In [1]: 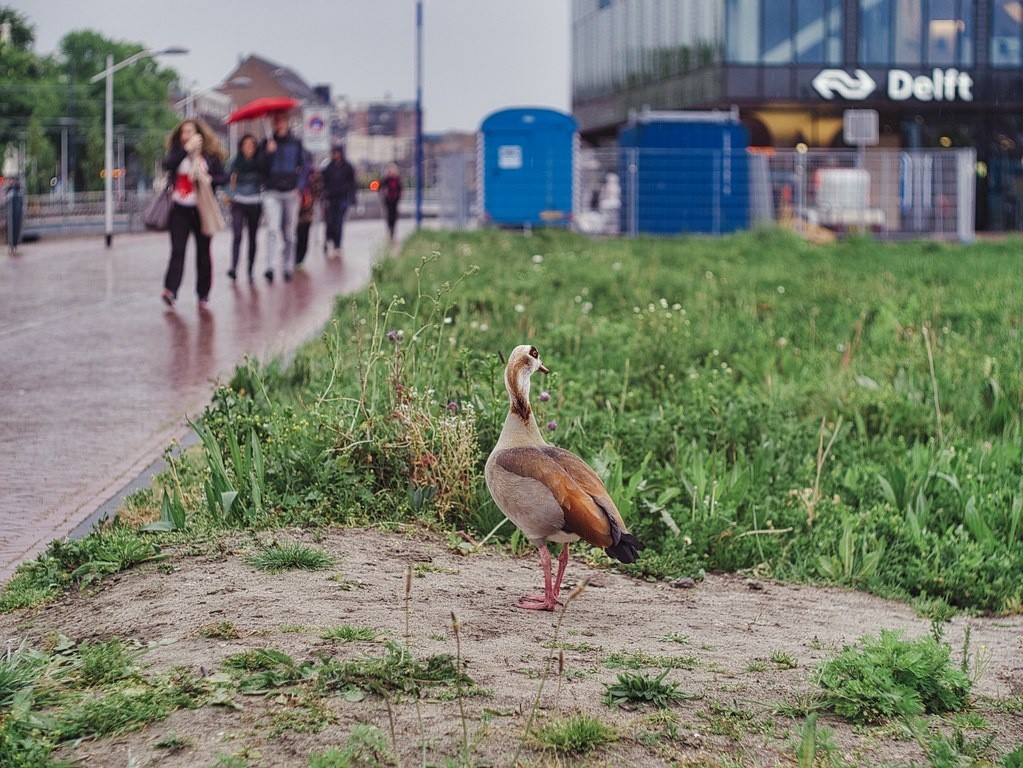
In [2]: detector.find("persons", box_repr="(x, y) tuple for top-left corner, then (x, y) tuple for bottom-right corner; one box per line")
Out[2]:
(161, 119), (229, 310)
(224, 135), (265, 280)
(258, 110), (307, 282)
(295, 153), (323, 271)
(381, 160), (401, 237)
(321, 144), (357, 249)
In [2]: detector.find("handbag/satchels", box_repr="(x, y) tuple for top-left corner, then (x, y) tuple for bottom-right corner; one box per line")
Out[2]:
(144, 187), (173, 231)
(194, 168), (226, 236)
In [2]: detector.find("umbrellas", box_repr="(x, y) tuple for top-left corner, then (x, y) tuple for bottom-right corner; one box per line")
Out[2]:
(225, 97), (299, 141)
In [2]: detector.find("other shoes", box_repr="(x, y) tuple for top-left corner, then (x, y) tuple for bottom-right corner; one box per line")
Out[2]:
(264, 269), (273, 283)
(163, 292), (177, 308)
(227, 267), (237, 280)
(199, 297), (208, 311)
(283, 271), (293, 283)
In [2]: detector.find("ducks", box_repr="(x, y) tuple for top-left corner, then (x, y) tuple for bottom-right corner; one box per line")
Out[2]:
(486, 345), (647, 612)
